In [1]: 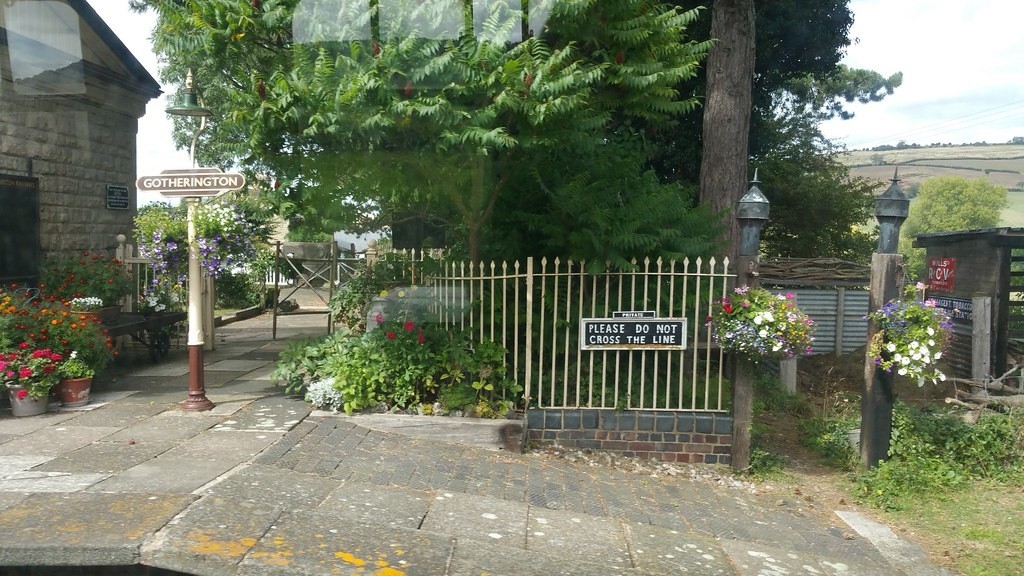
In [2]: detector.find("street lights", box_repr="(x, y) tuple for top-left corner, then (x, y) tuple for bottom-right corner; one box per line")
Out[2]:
(165, 68), (215, 413)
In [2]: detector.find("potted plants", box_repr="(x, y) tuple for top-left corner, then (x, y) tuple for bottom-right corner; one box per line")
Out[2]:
(53, 356), (96, 408)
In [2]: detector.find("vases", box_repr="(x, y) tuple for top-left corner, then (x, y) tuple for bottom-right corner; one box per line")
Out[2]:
(5, 378), (51, 417)
(98, 304), (125, 325)
(70, 308), (104, 326)
(140, 307), (165, 319)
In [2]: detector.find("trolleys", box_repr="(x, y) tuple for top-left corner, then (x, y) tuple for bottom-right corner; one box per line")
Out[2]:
(104, 310), (187, 364)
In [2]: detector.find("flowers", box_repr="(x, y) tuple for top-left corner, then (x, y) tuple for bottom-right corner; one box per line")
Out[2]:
(69, 296), (105, 312)
(0, 341), (64, 401)
(863, 280), (961, 387)
(705, 282), (818, 363)
(133, 278), (185, 313)
(65, 252), (138, 306)
